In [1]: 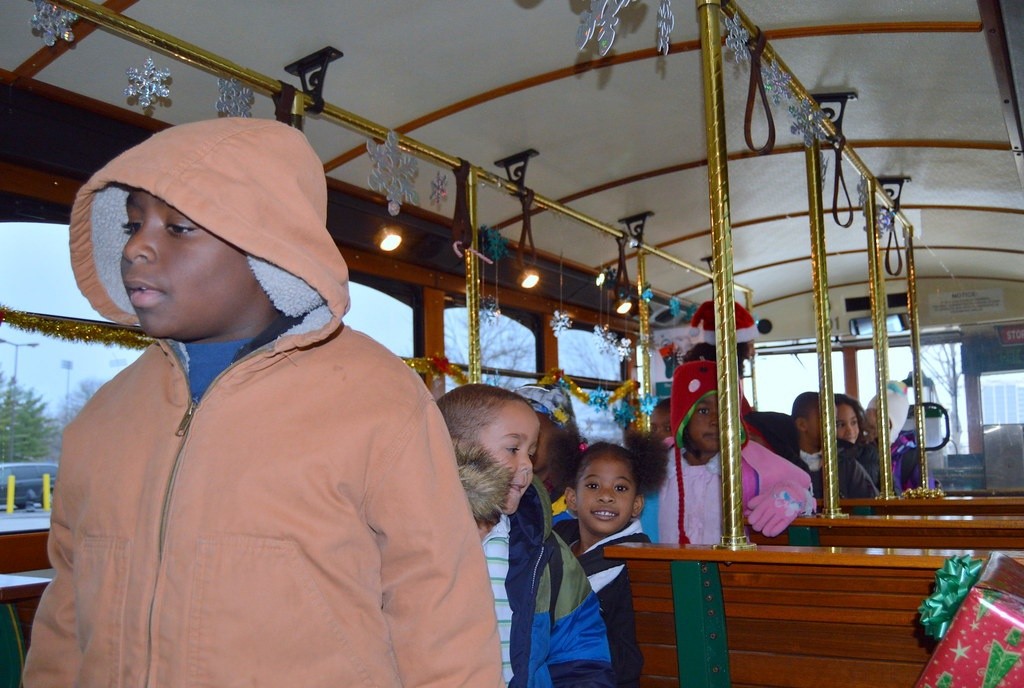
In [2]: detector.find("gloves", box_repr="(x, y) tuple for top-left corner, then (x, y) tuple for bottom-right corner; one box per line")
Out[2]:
(747, 486), (812, 537)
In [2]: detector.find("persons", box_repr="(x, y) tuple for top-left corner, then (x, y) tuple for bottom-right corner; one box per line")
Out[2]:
(689, 299), (758, 414)
(550, 440), (652, 688)
(511, 382), (587, 523)
(642, 397), (674, 441)
(789, 389), (879, 501)
(834, 393), (902, 497)
(641, 361), (815, 546)
(865, 381), (936, 493)
(435, 381), (611, 688)
(19, 118), (506, 688)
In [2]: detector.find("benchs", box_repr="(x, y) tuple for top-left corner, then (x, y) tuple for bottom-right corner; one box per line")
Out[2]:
(603, 542), (1024, 688)
(817, 495), (1024, 522)
(744, 513), (1024, 551)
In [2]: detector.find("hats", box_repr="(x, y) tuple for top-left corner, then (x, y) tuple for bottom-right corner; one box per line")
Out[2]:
(868, 379), (910, 445)
(670, 361), (749, 547)
(515, 383), (576, 432)
